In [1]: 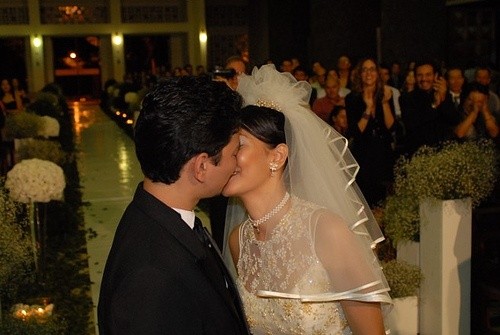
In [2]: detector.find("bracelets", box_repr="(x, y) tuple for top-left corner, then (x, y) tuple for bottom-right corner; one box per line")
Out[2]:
(484, 114), (496, 122)
(361, 112), (372, 120)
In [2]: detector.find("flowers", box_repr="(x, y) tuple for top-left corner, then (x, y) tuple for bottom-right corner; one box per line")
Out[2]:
(37, 116), (60, 140)
(4, 157), (67, 271)
(125, 92), (140, 105)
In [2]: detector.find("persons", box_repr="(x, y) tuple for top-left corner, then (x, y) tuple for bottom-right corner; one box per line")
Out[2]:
(122, 55), (500, 230)
(98, 76), (252, 335)
(0, 76), (26, 176)
(220, 64), (399, 335)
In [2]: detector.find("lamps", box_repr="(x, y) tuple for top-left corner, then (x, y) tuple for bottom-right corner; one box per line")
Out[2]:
(13, 303), (54, 325)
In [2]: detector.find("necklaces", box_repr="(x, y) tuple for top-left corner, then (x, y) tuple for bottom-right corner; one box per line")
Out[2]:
(248, 192), (290, 233)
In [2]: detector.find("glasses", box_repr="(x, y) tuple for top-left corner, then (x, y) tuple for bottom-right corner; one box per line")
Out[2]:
(362, 67), (377, 72)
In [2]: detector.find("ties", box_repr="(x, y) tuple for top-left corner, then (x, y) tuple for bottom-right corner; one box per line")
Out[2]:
(193, 216), (246, 323)
(454, 96), (459, 107)
